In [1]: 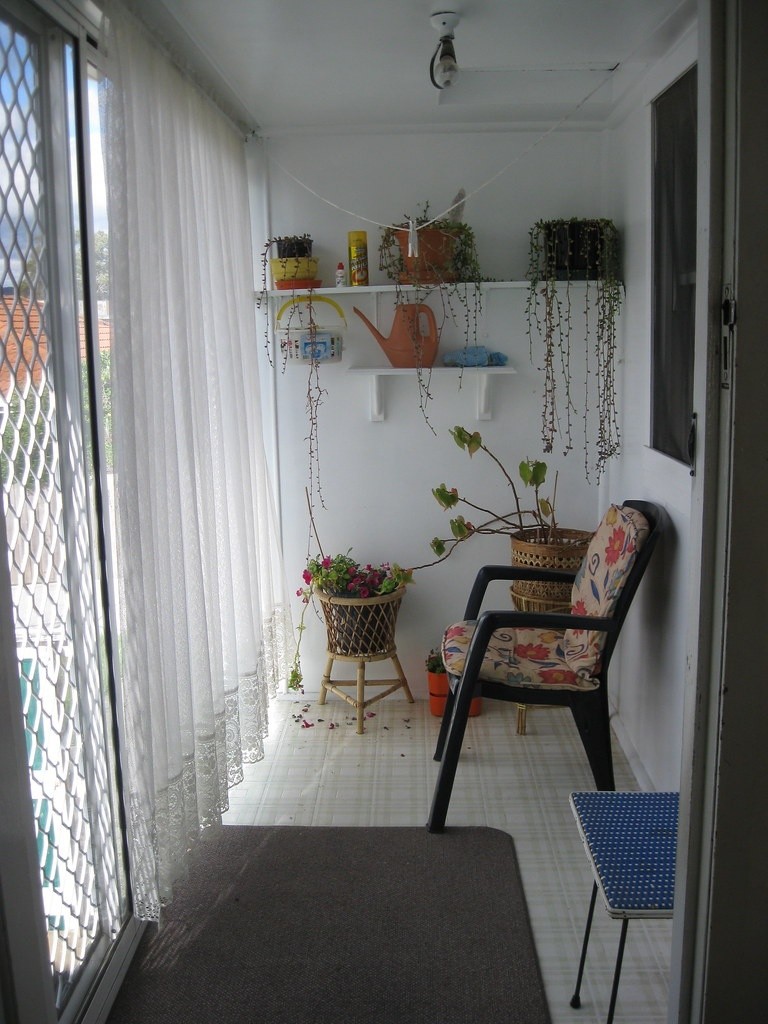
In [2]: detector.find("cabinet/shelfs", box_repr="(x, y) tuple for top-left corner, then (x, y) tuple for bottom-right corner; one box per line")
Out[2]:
(254, 278), (624, 423)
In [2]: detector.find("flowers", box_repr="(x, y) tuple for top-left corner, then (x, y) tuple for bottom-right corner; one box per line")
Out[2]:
(287, 546), (416, 690)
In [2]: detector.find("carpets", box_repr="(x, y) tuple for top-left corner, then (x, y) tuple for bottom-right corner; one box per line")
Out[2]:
(106, 825), (552, 1024)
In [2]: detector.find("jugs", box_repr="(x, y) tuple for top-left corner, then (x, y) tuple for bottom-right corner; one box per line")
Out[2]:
(350, 303), (438, 368)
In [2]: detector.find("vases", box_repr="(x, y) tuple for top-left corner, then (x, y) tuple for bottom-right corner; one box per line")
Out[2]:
(327, 594), (389, 654)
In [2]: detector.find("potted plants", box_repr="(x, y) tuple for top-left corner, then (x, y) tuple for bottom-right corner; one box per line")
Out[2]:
(425, 647), (481, 717)
(525, 216), (621, 485)
(256, 233), (328, 625)
(378, 200), (515, 437)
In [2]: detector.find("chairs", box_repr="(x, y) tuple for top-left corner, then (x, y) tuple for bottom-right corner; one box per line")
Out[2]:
(424, 500), (663, 833)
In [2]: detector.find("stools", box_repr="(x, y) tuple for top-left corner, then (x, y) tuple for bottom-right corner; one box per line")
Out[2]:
(568, 790), (679, 1024)
